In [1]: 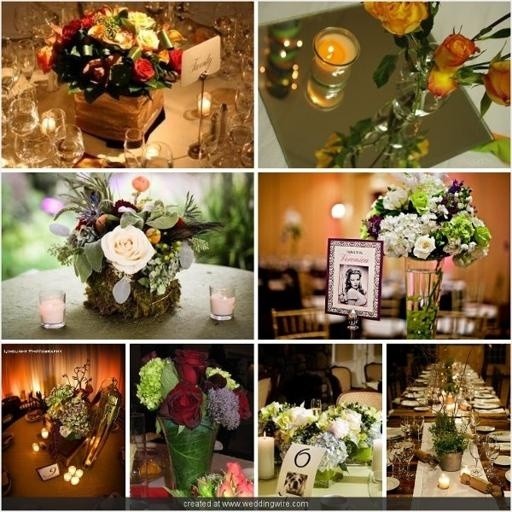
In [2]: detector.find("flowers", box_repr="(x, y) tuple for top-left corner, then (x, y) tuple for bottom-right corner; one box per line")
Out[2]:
(260, 399), (380, 484)
(39, 5), (184, 95)
(359, 171), (491, 270)
(41, 172), (225, 321)
(134, 347), (250, 428)
(311, 0), (508, 170)
(44, 360), (106, 441)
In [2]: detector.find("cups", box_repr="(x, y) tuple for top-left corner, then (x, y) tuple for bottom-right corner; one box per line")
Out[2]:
(209, 284), (237, 322)
(142, 2), (192, 33)
(303, 73), (345, 113)
(400, 400), (418, 406)
(304, 26), (361, 86)
(142, 140), (174, 168)
(414, 363), (434, 384)
(413, 406), (429, 411)
(405, 392), (422, 398)
(39, 289), (67, 330)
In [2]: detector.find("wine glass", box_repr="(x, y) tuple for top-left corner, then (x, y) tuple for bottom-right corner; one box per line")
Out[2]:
(402, 444), (415, 481)
(387, 445), (398, 478)
(486, 434), (498, 443)
(469, 441), (485, 473)
(400, 415), (414, 442)
(1, 2), (75, 98)
(39, 107), (67, 141)
(311, 399), (322, 416)
(198, 7), (254, 168)
(413, 415), (425, 443)
(53, 123), (85, 168)
(6, 98), (39, 137)
(484, 443), (500, 479)
(393, 442), (405, 473)
(13, 125), (53, 168)
(129, 411), (148, 488)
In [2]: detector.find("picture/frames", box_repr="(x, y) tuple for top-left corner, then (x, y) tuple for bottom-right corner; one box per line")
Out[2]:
(326, 238), (383, 322)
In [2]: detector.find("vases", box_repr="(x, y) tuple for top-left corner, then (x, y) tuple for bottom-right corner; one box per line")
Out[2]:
(43, 425), (87, 464)
(160, 414), (218, 497)
(71, 86), (166, 148)
(402, 270), (442, 341)
(384, 101), (426, 166)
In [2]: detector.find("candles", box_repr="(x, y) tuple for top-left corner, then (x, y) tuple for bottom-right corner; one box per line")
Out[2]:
(437, 473), (450, 490)
(198, 92), (211, 115)
(258, 430), (276, 479)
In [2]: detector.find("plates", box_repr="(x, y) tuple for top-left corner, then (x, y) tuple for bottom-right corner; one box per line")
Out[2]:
(460, 363), (485, 384)
(489, 430), (510, 442)
(476, 425), (496, 432)
(489, 442), (510, 452)
(472, 403), (500, 409)
(505, 469), (511, 482)
(387, 477), (400, 492)
(471, 385), (500, 403)
(386, 426), (410, 438)
(493, 455), (511, 465)
(406, 386), (427, 392)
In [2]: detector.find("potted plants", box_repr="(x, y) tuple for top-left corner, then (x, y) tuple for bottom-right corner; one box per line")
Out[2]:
(429, 411), (477, 470)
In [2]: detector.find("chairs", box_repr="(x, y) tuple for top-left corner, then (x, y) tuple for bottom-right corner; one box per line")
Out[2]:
(486, 363), (494, 386)
(387, 387), (393, 409)
(433, 310), (465, 339)
(336, 391), (380, 412)
(500, 378), (509, 406)
(331, 366), (352, 390)
(395, 380), (401, 397)
(270, 307), (330, 339)
(365, 362), (381, 383)
(360, 299), (400, 337)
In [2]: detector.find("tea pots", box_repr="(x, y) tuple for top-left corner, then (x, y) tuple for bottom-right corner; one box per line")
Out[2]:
(79, 377), (123, 471)
